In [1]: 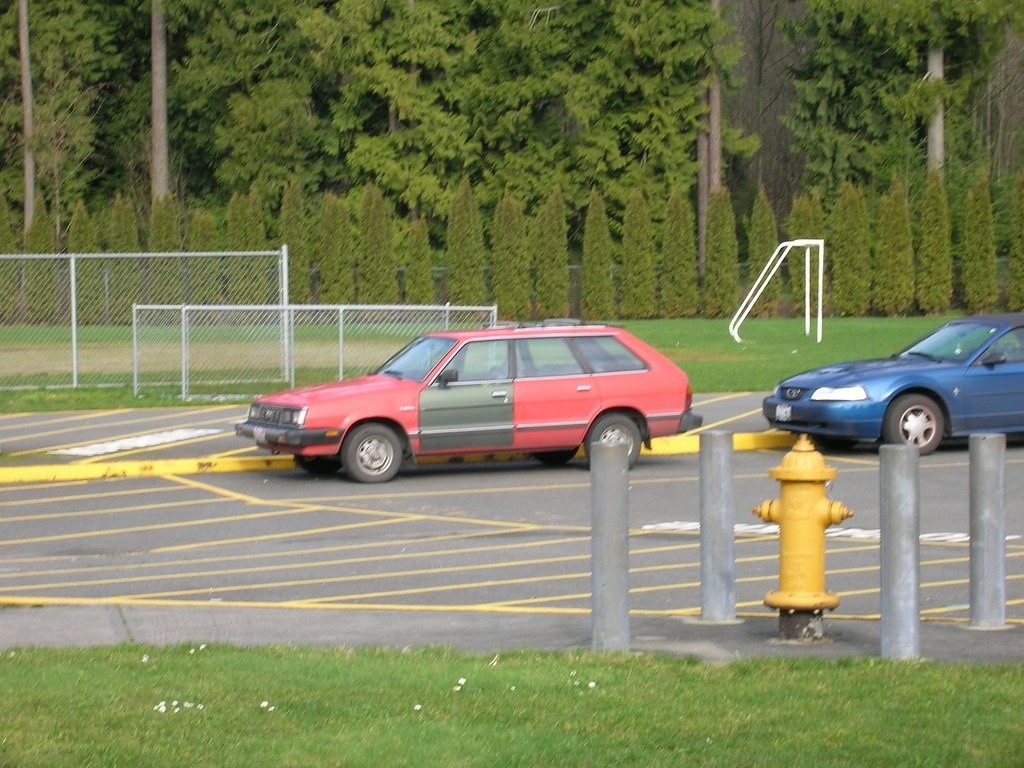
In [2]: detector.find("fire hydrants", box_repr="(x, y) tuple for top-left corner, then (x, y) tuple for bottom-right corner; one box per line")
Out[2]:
(747, 430), (854, 640)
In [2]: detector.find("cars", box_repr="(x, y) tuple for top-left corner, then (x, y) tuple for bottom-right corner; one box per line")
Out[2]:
(761, 313), (1024, 456)
(238, 324), (703, 482)
